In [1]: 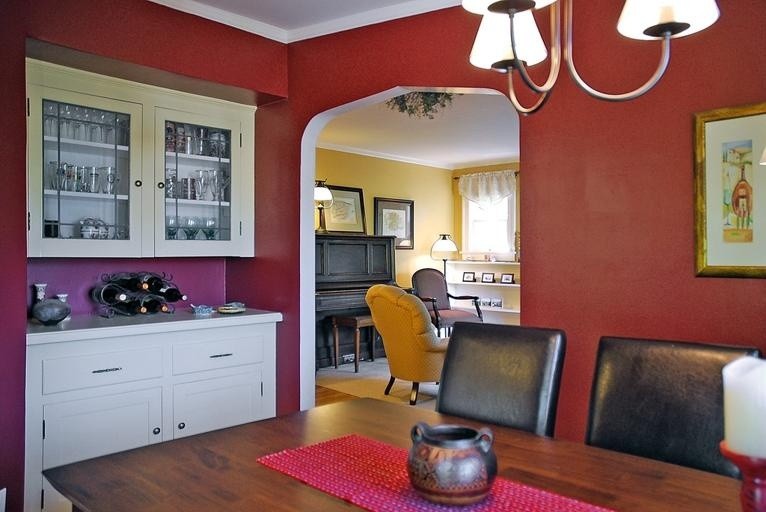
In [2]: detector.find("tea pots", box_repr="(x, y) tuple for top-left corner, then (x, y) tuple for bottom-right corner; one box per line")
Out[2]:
(80, 216), (108, 239)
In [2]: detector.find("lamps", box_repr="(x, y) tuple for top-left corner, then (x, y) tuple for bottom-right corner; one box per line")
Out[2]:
(462, 0), (721, 115)
(314, 180), (333, 234)
(430, 234), (459, 276)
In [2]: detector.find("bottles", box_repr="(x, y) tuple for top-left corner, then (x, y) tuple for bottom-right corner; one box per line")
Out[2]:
(166, 216), (222, 239)
(89, 273), (190, 314)
(33, 282), (72, 322)
(165, 125), (227, 157)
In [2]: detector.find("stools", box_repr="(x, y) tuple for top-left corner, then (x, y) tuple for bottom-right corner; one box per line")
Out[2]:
(332, 311), (376, 373)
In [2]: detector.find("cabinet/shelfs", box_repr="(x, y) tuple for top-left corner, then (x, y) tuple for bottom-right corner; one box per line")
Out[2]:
(24, 56), (255, 260)
(24, 323), (278, 512)
(447, 261), (521, 327)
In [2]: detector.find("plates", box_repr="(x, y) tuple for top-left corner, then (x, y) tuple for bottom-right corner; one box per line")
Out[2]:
(192, 306), (247, 314)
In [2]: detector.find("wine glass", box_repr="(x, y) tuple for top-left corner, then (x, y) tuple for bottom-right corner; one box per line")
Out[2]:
(43, 102), (130, 146)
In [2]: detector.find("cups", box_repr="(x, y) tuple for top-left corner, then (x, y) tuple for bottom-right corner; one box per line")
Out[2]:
(45, 219), (59, 238)
(166, 169), (224, 202)
(51, 161), (120, 195)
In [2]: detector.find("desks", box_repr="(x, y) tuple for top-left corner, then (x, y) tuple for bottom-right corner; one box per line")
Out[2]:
(41, 395), (742, 512)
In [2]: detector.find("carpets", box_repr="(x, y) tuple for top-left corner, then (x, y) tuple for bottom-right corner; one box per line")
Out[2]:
(315, 356), (438, 405)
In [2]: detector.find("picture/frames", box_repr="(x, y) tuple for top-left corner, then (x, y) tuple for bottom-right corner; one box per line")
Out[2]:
(500, 274), (515, 284)
(481, 273), (495, 283)
(693, 101), (766, 279)
(322, 184), (368, 236)
(463, 272), (476, 282)
(374, 197), (414, 250)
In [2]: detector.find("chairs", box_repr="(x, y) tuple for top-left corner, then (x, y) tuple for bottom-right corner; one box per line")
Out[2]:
(435, 318), (566, 441)
(585, 335), (762, 483)
(365, 283), (450, 406)
(410, 268), (483, 338)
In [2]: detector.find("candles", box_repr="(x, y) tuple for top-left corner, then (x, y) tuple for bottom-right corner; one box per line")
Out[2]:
(722, 355), (766, 458)
